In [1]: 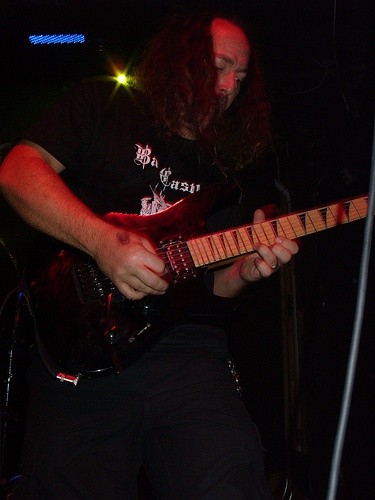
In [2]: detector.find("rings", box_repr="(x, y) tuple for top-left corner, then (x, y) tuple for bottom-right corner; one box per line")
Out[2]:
(271, 263), (278, 269)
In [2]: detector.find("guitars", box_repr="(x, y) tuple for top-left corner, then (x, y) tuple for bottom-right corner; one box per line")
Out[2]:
(21, 191), (375, 388)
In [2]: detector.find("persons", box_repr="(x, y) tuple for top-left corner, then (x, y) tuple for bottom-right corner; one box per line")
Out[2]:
(1, 4), (300, 500)
